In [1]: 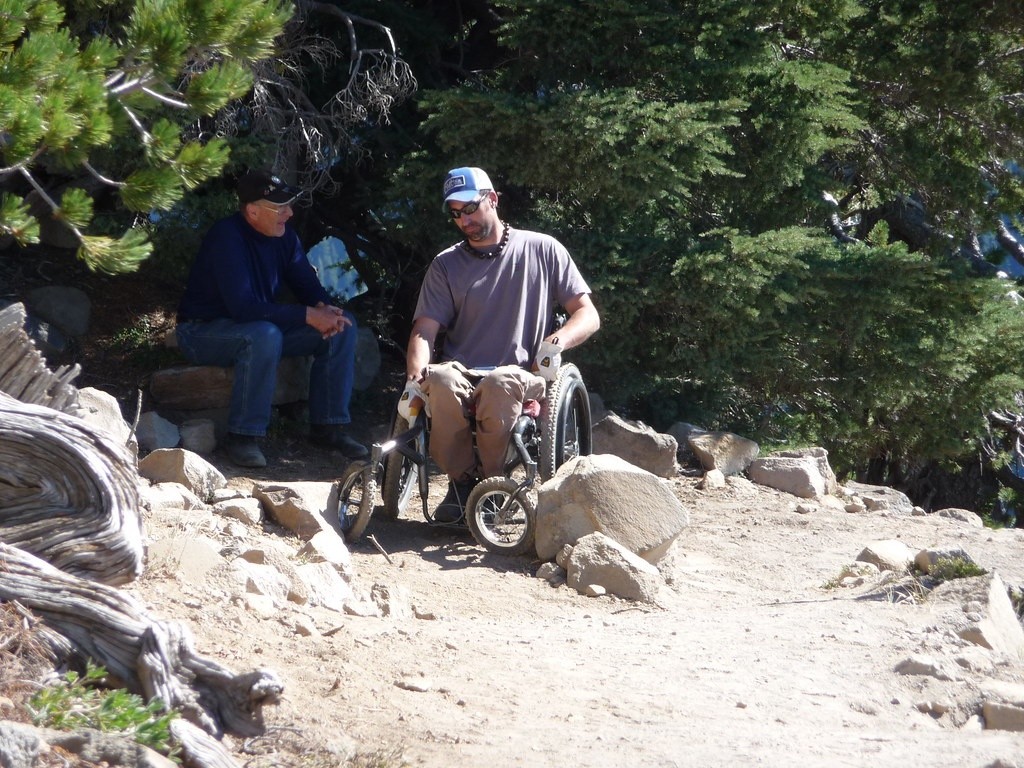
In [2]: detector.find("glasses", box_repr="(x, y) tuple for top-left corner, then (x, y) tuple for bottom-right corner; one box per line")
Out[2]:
(256, 203), (288, 215)
(450, 193), (488, 219)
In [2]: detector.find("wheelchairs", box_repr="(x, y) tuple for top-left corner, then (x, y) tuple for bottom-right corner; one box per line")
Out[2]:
(337, 310), (595, 558)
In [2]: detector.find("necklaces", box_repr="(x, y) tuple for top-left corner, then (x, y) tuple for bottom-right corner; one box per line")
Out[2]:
(463, 220), (510, 260)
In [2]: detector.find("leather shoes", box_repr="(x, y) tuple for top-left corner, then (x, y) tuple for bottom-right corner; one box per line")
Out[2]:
(309, 424), (369, 457)
(226, 432), (267, 467)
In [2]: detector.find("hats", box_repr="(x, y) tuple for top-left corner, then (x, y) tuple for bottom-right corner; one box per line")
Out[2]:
(441, 166), (494, 213)
(237, 169), (305, 205)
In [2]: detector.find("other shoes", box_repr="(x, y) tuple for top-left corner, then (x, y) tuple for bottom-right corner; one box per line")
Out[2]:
(481, 492), (504, 523)
(434, 464), (484, 522)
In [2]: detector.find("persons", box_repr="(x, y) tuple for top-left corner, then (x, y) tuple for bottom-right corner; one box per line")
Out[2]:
(399, 165), (602, 525)
(174, 170), (370, 468)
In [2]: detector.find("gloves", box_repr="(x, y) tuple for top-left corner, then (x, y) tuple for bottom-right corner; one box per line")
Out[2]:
(397, 376), (431, 430)
(530, 337), (563, 381)
